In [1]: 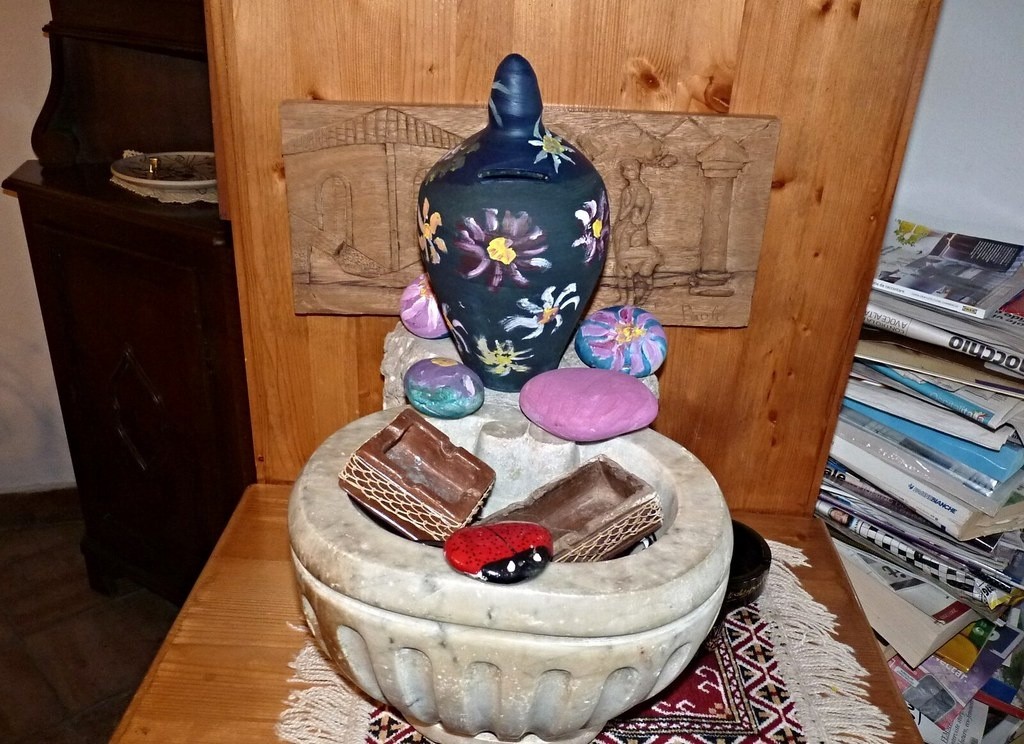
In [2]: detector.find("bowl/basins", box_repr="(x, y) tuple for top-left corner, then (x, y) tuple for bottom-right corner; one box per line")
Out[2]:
(717, 519), (770, 627)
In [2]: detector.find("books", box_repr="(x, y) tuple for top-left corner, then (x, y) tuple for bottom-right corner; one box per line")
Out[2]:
(812, 214), (1024, 744)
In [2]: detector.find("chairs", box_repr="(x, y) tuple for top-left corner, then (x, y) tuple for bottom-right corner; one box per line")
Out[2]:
(109, 0), (946, 744)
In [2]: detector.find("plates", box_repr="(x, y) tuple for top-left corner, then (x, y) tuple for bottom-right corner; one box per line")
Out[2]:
(111, 152), (217, 190)
